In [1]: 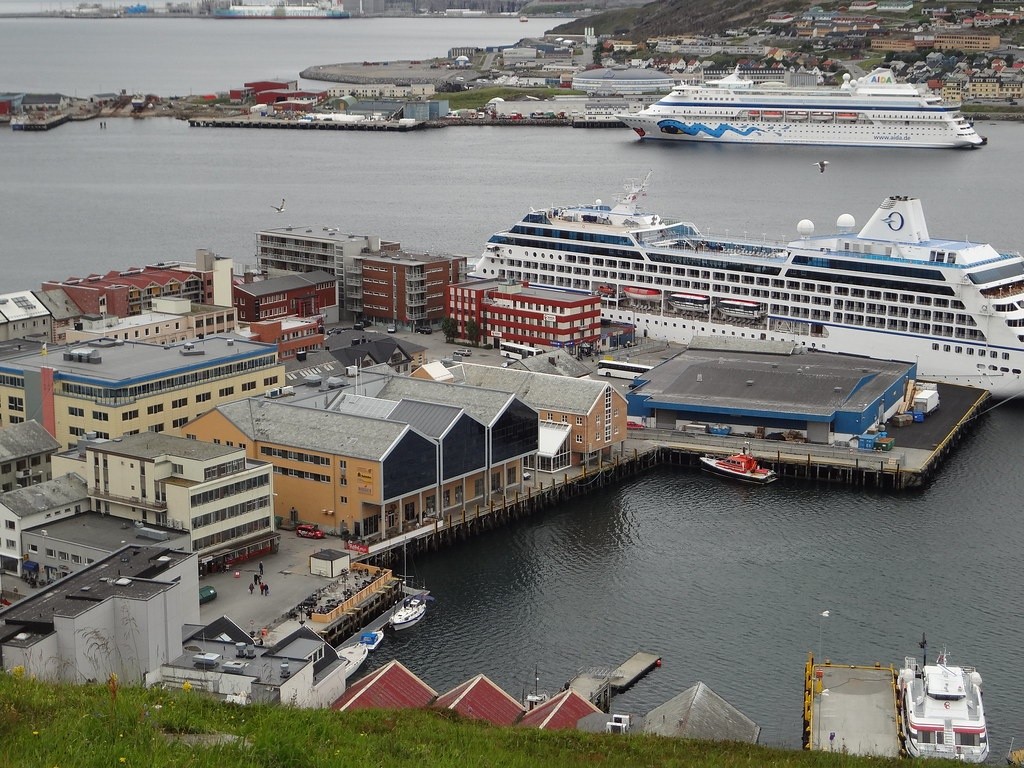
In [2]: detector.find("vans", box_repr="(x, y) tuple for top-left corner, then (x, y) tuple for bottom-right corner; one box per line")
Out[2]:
(387, 324), (397, 333)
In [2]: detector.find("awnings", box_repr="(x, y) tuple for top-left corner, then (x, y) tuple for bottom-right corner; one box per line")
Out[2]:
(22, 561), (39, 573)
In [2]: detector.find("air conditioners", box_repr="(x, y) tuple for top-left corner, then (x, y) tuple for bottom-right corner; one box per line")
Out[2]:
(605, 721), (626, 735)
(613, 714), (629, 729)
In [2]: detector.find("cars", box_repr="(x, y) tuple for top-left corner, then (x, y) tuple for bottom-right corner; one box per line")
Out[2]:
(357, 320), (372, 328)
(626, 421), (645, 430)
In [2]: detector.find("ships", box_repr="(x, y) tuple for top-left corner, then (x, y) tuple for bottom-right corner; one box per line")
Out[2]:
(613, 63), (984, 150)
(215, 4), (351, 20)
(465, 168), (1024, 401)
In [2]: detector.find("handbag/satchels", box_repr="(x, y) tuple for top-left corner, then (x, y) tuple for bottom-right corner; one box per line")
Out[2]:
(248, 589), (251, 593)
(266, 591), (269, 594)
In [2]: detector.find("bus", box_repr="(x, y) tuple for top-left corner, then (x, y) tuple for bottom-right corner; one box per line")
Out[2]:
(500, 341), (543, 361)
(597, 360), (654, 380)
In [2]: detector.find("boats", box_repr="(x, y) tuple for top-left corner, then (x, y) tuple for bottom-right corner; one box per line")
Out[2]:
(597, 285), (616, 295)
(761, 112), (783, 118)
(810, 112), (833, 120)
(336, 643), (369, 680)
(836, 113), (858, 120)
(786, 111), (807, 119)
(667, 293), (710, 313)
(360, 630), (384, 653)
(715, 300), (761, 320)
(623, 286), (661, 301)
(390, 599), (427, 631)
(900, 649), (990, 761)
(748, 111), (759, 117)
(699, 452), (778, 487)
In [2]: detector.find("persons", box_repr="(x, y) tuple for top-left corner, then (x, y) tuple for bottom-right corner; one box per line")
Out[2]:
(249, 627), (268, 646)
(416, 510), (428, 524)
(341, 526), (350, 550)
(217, 562), (232, 573)
(295, 567), (379, 620)
(14, 577), (53, 593)
(248, 560), (268, 596)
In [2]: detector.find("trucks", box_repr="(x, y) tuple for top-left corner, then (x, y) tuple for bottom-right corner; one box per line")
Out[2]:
(914, 390), (941, 417)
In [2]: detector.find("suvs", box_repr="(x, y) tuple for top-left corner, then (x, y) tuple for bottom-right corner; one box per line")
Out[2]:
(453, 348), (472, 357)
(415, 327), (432, 335)
(296, 524), (324, 540)
